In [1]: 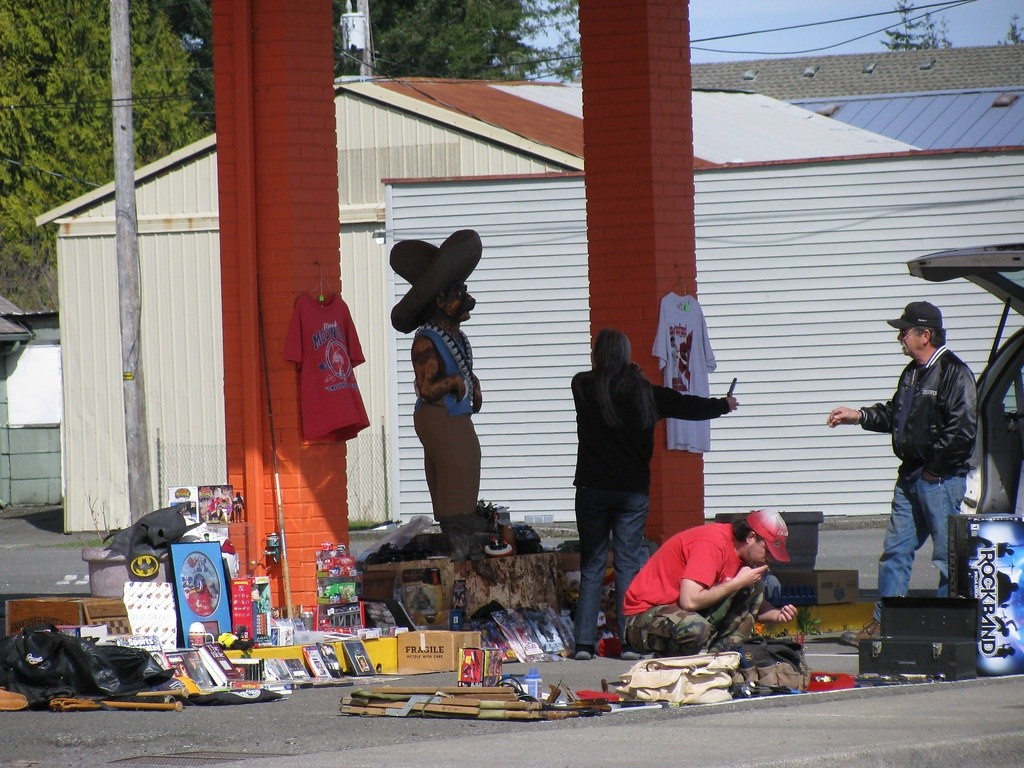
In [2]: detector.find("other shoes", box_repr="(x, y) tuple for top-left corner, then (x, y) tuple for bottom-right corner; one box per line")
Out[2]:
(619, 647), (655, 659)
(574, 644), (597, 659)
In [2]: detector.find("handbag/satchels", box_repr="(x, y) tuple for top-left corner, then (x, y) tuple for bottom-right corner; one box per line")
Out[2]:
(1, 623), (183, 701)
(614, 632), (812, 705)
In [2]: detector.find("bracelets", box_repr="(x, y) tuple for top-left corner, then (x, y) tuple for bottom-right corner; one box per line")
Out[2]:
(854, 410), (860, 425)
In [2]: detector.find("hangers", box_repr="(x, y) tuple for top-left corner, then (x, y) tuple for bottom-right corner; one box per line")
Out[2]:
(307, 260), (336, 296)
(671, 264), (690, 296)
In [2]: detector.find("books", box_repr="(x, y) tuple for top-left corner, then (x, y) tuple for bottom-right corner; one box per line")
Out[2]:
(197, 644), (242, 686)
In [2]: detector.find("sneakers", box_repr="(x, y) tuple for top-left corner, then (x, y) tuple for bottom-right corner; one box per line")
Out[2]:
(840, 618), (881, 647)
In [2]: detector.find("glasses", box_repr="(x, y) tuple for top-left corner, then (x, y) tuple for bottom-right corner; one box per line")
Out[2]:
(902, 329), (914, 336)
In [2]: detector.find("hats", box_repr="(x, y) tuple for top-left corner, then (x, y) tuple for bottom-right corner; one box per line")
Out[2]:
(887, 300), (942, 328)
(748, 508), (791, 561)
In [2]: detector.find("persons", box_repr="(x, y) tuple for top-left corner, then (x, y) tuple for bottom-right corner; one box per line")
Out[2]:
(388, 229), (484, 517)
(357, 657), (370, 671)
(620, 510), (798, 659)
(826, 300), (978, 637)
(571, 330), (739, 660)
(319, 644), (342, 677)
(274, 659), (284, 675)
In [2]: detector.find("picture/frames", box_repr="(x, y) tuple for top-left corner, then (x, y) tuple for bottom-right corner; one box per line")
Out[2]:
(167, 541), (234, 648)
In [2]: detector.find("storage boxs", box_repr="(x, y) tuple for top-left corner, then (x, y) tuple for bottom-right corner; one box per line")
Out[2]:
(397, 630), (481, 672)
(858, 596), (981, 680)
(4, 597), (123, 645)
(414, 609), (463, 631)
(317, 557), (383, 639)
(168, 484), (235, 525)
(230, 576), (295, 647)
(458, 646), (502, 687)
(294, 631), (324, 644)
(769, 569), (859, 606)
(802, 642), (860, 675)
(947, 514), (1024, 677)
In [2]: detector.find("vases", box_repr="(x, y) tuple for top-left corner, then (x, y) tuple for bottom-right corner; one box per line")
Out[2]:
(715, 512), (824, 569)
(82, 547), (173, 597)
